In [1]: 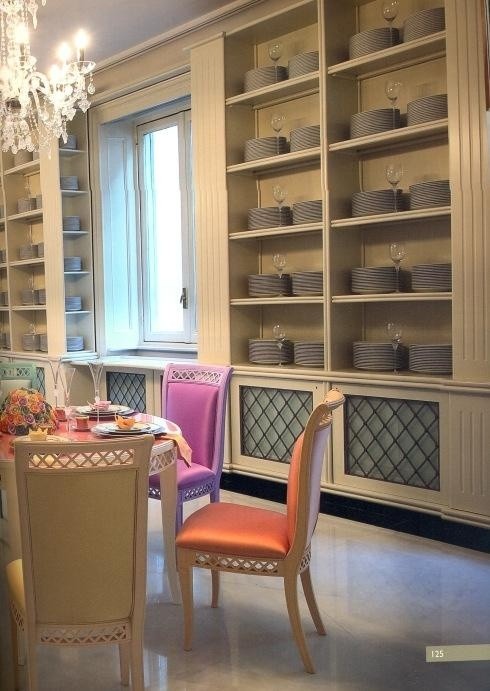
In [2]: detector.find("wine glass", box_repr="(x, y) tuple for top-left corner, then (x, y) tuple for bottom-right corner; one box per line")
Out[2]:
(49, 357), (104, 430)
(268, 42), (288, 369)
(384, 1), (407, 374)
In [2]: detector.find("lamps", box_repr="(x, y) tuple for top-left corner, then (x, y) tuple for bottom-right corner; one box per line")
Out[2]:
(0, 0), (96, 155)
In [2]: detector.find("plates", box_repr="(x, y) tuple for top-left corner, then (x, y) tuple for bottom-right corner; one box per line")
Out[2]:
(8, 434), (70, 452)
(246, 337), (326, 368)
(71, 404), (136, 418)
(347, 179), (451, 215)
(242, 125), (324, 161)
(345, 263), (454, 294)
(348, 95), (447, 141)
(0, 135), (85, 354)
(246, 270), (325, 298)
(354, 340), (451, 374)
(342, 6), (446, 59)
(237, 200), (325, 229)
(243, 50), (320, 91)
(94, 421), (167, 436)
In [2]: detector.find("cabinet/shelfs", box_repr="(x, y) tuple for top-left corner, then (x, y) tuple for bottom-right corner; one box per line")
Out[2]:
(228, 375), (447, 514)
(0, 109), (97, 361)
